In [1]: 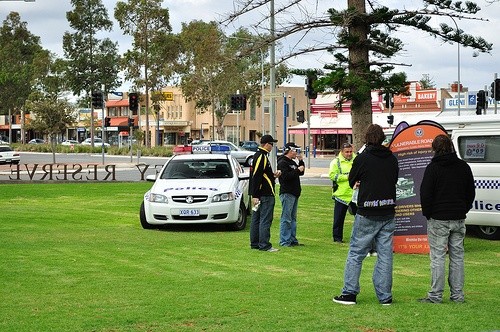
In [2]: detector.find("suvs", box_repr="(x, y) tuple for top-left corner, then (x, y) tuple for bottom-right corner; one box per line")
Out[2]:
(201, 141), (257, 168)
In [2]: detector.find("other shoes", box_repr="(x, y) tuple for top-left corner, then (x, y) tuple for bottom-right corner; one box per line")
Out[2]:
(295, 243), (304, 246)
(418, 298), (434, 304)
(286, 244), (293, 247)
(266, 247), (279, 252)
(334, 240), (343, 243)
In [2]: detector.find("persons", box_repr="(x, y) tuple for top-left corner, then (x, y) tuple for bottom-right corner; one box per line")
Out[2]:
(420, 133), (475, 302)
(329, 143), (357, 243)
(277, 143), (306, 246)
(248, 135), (281, 251)
(332, 124), (399, 305)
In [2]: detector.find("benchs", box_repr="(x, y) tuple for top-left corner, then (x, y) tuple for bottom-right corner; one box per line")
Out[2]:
(191, 166), (216, 176)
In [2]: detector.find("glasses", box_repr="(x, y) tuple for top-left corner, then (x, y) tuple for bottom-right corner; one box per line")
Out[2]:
(265, 143), (273, 146)
(292, 150), (297, 152)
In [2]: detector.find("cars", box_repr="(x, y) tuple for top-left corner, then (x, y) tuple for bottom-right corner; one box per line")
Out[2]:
(82, 137), (110, 147)
(0, 146), (20, 164)
(139, 146), (251, 231)
(241, 141), (259, 152)
(28, 139), (45, 144)
(61, 140), (80, 145)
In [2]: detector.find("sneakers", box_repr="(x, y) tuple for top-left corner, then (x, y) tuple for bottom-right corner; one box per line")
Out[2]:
(333, 294), (356, 304)
(382, 298), (392, 305)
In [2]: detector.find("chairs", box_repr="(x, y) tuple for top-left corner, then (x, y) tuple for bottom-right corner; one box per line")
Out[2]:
(176, 164), (190, 174)
(214, 164), (228, 176)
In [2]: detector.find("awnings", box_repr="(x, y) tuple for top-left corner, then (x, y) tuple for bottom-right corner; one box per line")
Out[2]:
(86, 117), (138, 131)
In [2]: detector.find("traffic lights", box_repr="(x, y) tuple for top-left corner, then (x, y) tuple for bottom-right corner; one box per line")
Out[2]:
(129, 93), (138, 110)
(230, 95), (248, 110)
(491, 78), (500, 101)
(476, 90), (485, 114)
(297, 110), (305, 122)
(128, 118), (134, 126)
(92, 92), (103, 109)
(307, 75), (318, 99)
(387, 115), (394, 124)
(105, 117), (111, 127)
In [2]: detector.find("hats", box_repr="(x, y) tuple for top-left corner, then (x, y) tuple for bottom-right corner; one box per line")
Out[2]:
(260, 135), (278, 143)
(284, 142), (301, 150)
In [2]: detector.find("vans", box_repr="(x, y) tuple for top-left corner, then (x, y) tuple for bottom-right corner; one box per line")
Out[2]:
(450, 128), (500, 240)
